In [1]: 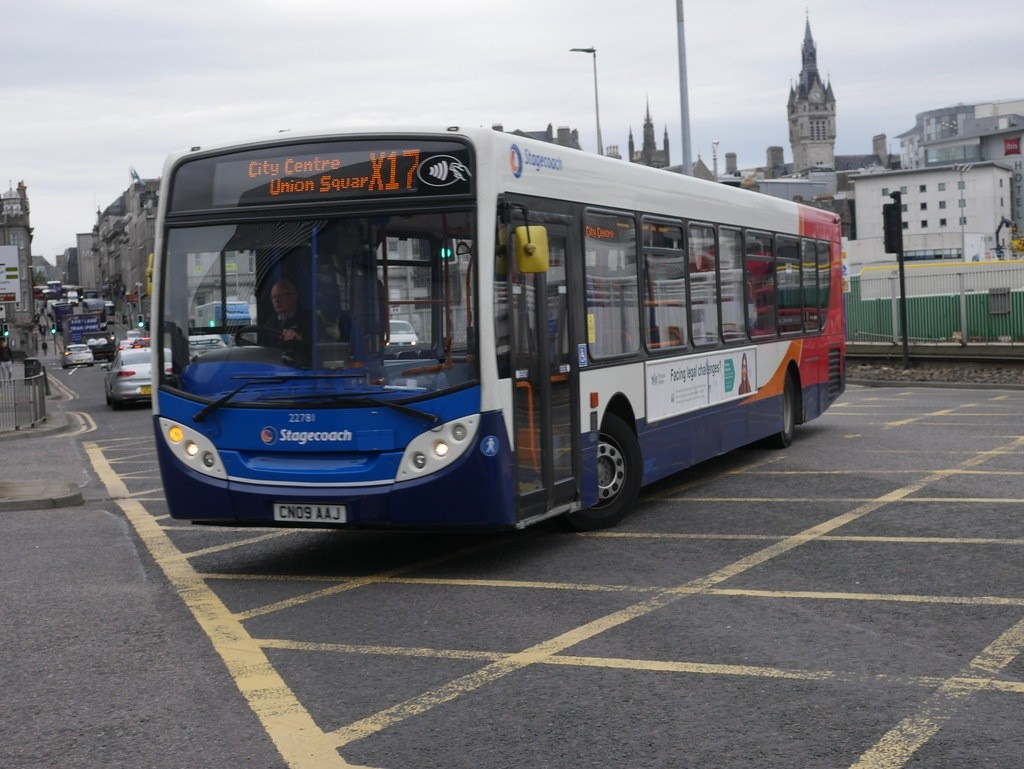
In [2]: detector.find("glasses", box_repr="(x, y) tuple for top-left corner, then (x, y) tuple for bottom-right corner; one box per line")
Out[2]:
(270, 293), (293, 298)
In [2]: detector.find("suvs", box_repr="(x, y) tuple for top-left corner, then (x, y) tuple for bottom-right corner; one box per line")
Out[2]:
(80, 332), (115, 362)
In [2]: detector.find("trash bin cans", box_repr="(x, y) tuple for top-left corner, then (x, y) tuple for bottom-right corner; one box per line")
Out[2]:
(123, 316), (127, 324)
(24, 358), (41, 385)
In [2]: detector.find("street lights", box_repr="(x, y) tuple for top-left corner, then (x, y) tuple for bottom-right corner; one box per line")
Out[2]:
(569, 46), (604, 155)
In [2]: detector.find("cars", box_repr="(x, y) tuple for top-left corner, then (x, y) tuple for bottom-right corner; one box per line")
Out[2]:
(104, 329), (177, 410)
(60, 344), (95, 368)
(384, 319), (419, 346)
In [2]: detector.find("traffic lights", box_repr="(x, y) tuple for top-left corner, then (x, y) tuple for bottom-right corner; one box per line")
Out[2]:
(3, 323), (9, 337)
(137, 314), (144, 329)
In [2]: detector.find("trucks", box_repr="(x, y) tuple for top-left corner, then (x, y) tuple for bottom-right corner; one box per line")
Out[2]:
(194, 300), (253, 328)
(32, 280), (116, 348)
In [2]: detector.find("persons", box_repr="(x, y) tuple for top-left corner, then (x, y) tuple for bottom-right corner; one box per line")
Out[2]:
(346, 247), (390, 383)
(0, 340), (15, 386)
(261, 279), (323, 365)
(42, 342), (48, 356)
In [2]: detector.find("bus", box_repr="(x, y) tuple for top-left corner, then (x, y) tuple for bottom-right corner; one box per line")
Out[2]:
(145, 130), (847, 543)
